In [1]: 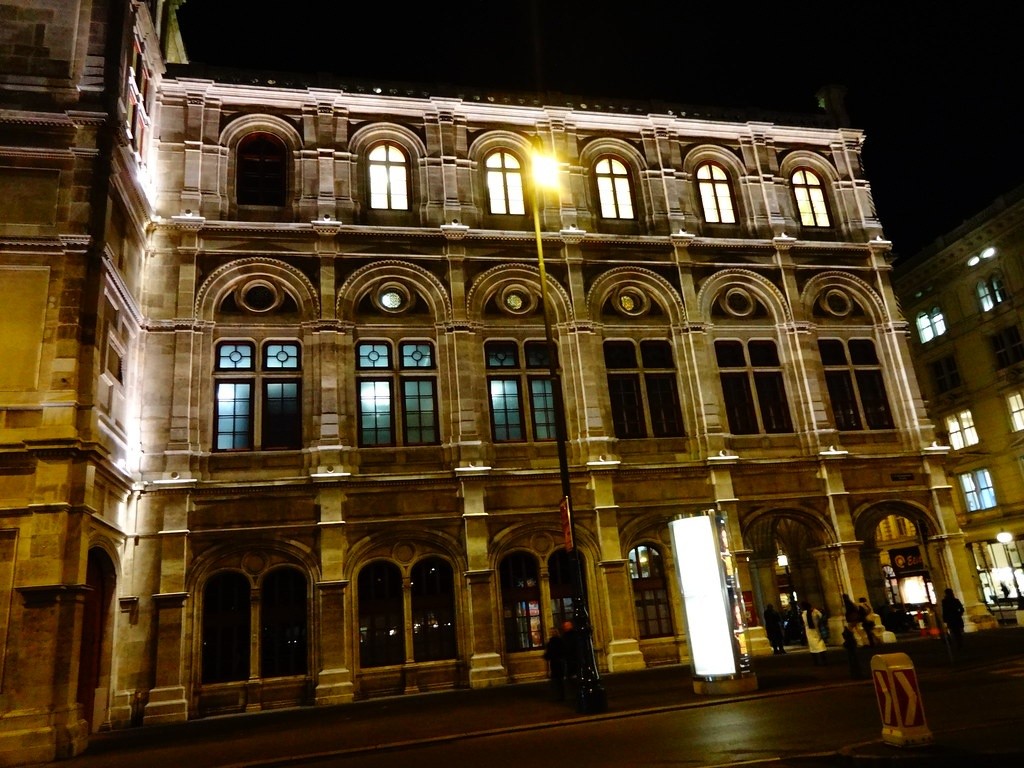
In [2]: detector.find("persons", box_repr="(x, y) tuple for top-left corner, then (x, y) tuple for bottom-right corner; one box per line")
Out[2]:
(842, 593), (875, 648)
(801, 602), (829, 665)
(1001, 584), (1009, 598)
(941, 588), (964, 656)
(764, 604), (787, 654)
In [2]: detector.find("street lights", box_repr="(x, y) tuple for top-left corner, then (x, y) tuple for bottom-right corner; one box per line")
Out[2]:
(996, 531), (1024, 610)
(524, 151), (607, 715)
